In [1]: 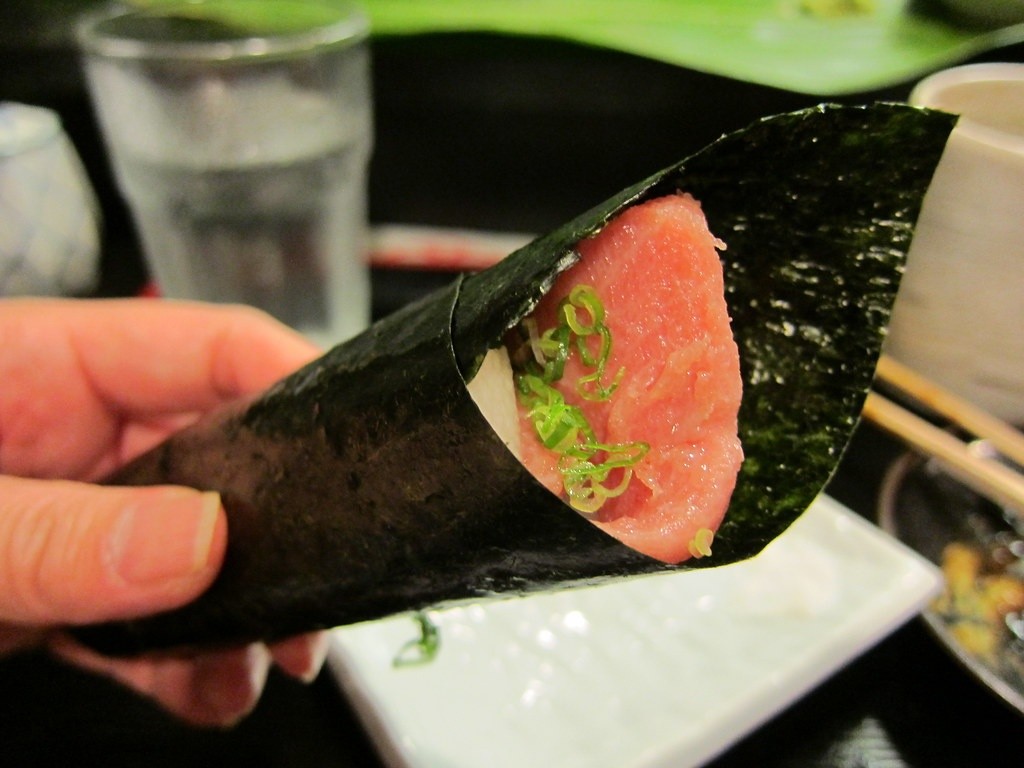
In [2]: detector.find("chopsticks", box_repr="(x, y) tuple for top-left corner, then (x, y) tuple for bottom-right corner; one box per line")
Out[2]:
(859, 351), (1023, 514)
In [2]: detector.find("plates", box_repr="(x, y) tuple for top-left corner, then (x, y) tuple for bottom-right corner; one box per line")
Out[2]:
(880, 426), (1023, 715)
(321, 490), (945, 768)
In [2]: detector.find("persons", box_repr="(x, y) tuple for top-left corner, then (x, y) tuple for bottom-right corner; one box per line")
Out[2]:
(1, 288), (336, 735)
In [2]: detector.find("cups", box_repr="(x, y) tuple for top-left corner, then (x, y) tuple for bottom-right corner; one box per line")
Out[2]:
(890, 59), (1023, 428)
(3, 98), (96, 303)
(74, 0), (377, 358)
